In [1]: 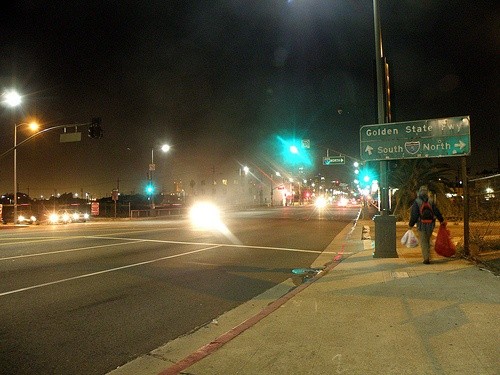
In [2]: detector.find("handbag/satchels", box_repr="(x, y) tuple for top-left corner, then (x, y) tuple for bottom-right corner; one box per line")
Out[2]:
(434, 222), (456, 258)
(401, 230), (419, 248)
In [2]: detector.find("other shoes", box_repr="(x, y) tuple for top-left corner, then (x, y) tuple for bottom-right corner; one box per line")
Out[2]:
(423, 261), (430, 264)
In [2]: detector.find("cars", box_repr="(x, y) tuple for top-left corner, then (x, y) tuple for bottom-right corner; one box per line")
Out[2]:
(15, 211), (36, 226)
(45, 208), (89, 225)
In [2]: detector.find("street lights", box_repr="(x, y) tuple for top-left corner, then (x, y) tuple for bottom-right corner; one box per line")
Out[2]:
(14, 121), (38, 224)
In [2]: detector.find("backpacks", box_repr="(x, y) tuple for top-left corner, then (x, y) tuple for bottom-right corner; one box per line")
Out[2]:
(417, 201), (435, 230)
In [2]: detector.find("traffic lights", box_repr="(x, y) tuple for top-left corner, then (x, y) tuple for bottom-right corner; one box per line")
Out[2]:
(88, 127), (96, 140)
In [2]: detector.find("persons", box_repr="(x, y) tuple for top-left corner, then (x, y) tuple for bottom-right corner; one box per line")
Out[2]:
(281, 192), (297, 207)
(408, 186), (447, 264)
